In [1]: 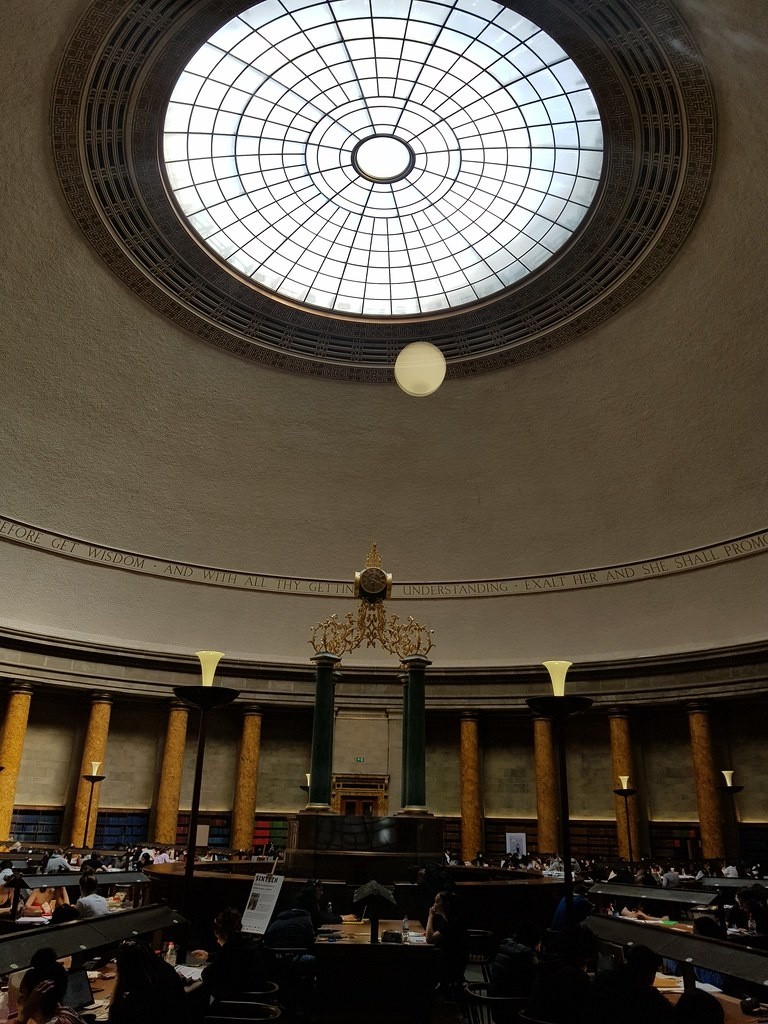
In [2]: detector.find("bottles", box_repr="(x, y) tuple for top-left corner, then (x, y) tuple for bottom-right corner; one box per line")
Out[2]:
(165, 942), (177, 967)
(402, 915), (410, 944)
(609, 903), (614, 916)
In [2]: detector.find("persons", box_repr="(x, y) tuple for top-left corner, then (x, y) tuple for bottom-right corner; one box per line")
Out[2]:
(108, 939), (194, 1024)
(0, 840), (768, 1024)
(5, 947), (89, 1024)
(190, 912), (273, 990)
(419, 891), (472, 973)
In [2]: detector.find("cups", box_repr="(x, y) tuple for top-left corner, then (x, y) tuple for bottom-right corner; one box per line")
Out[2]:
(614, 911), (619, 918)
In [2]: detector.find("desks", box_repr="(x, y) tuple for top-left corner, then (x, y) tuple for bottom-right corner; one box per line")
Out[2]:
(605, 908), (768, 1024)
(0, 861), (211, 1024)
(314, 918), (435, 1017)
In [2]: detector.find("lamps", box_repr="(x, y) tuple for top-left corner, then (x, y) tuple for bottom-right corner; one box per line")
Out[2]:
(171, 650), (242, 962)
(306, 773), (311, 786)
(718, 770), (743, 864)
(524, 660), (598, 927)
(614, 776), (635, 873)
(82, 761), (105, 848)
(394, 342), (447, 397)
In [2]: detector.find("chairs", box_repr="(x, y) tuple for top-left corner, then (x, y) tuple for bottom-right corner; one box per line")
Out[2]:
(462, 981), (558, 1024)
(204, 981), (281, 1024)
(467, 929), (496, 983)
(264, 946), (311, 1003)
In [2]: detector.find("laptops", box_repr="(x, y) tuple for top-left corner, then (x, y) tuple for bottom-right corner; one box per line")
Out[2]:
(341, 905), (367, 924)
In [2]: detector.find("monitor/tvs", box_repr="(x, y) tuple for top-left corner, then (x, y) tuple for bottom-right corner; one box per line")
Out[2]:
(61, 966), (95, 1012)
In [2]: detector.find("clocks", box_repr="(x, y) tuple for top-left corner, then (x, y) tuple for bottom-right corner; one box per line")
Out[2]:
(360, 568), (387, 594)
